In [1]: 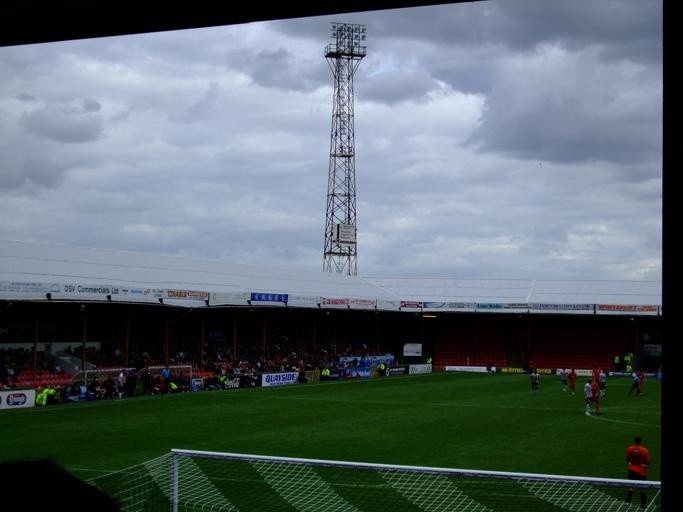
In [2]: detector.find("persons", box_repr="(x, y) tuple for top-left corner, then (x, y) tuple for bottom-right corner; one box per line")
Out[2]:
(0, 335), (389, 409)
(623, 436), (652, 512)
(529, 369), (540, 391)
(560, 351), (644, 419)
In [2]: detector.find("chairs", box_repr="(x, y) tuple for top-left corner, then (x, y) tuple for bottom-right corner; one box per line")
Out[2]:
(0, 359), (215, 391)
(430, 346), (613, 373)
